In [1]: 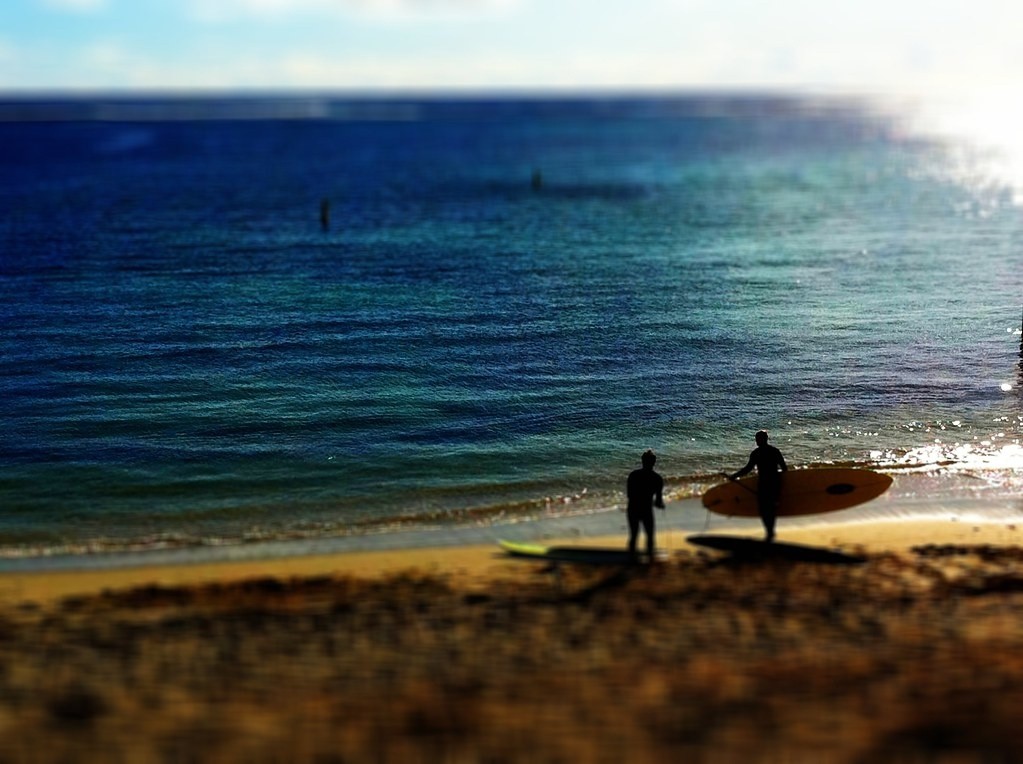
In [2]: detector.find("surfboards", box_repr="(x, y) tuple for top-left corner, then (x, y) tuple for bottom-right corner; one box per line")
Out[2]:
(703, 468), (893, 516)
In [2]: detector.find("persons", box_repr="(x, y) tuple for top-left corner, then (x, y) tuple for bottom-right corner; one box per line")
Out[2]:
(727, 431), (789, 542)
(625, 450), (667, 560)
